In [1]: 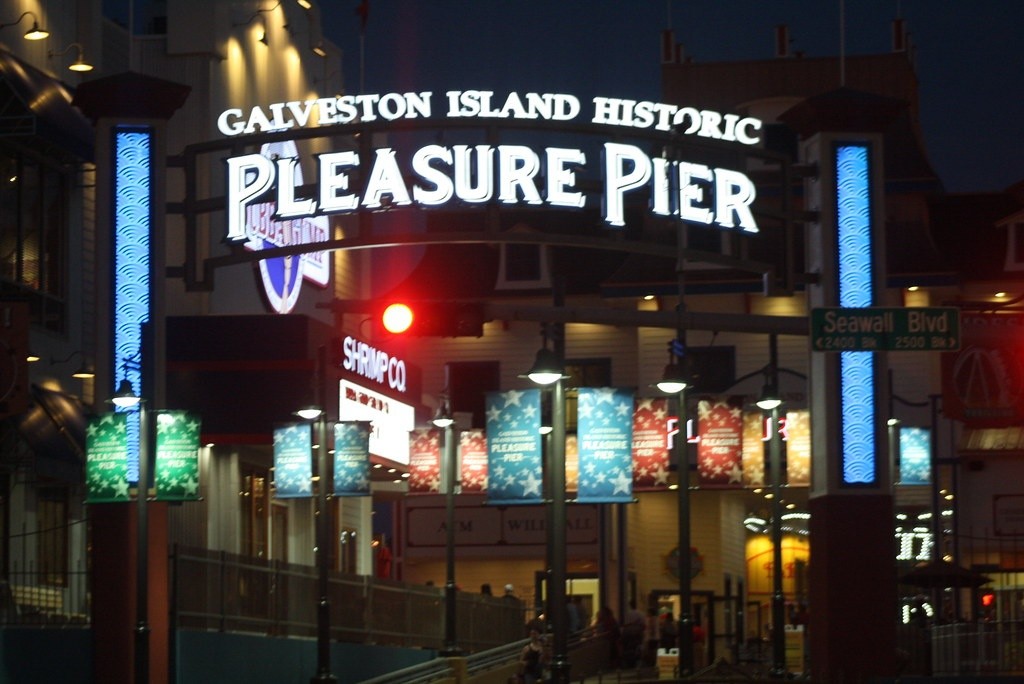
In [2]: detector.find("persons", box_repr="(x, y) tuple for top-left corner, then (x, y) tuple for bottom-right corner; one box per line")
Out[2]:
(502, 583), (518, 601)
(622, 600), (646, 672)
(787, 603), (810, 636)
(655, 604), (674, 654)
(425, 580), (434, 587)
(642, 605), (658, 664)
(565, 594), (588, 641)
(596, 605), (623, 674)
(517, 626), (549, 684)
(691, 619), (707, 673)
(480, 584), (493, 596)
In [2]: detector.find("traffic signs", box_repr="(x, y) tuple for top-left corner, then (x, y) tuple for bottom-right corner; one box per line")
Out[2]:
(810, 307), (962, 352)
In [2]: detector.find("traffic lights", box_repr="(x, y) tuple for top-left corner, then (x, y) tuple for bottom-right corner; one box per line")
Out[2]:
(379, 302), (483, 339)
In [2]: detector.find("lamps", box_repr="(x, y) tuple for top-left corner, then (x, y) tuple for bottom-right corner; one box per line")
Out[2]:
(648, 339), (695, 393)
(0, 11), (49, 40)
(103, 364), (149, 407)
(426, 390), (457, 427)
(515, 321), (572, 385)
(48, 43), (94, 71)
(51, 351), (97, 379)
(748, 365), (788, 410)
(291, 386), (329, 420)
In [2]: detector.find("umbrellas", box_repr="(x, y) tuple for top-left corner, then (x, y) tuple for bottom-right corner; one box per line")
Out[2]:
(899, 559), (994, 591)
